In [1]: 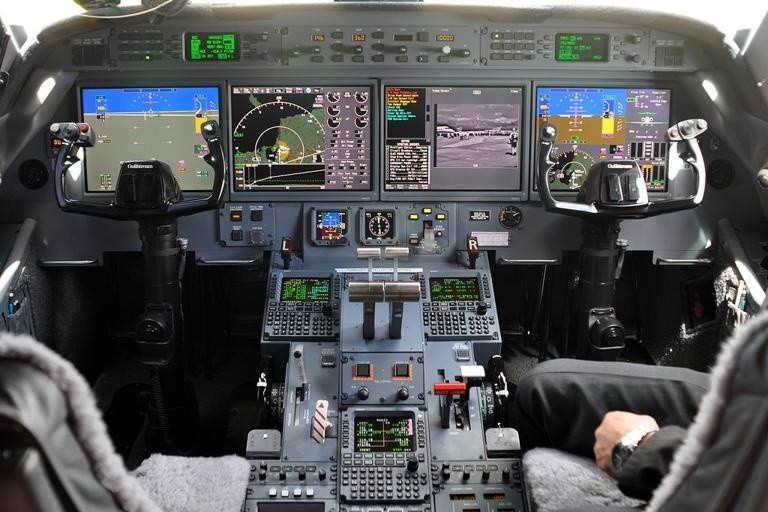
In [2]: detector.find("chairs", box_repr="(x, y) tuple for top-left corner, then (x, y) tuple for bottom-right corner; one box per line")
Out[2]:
(1, 334), (250, 512)
(521, 309), (767, 512)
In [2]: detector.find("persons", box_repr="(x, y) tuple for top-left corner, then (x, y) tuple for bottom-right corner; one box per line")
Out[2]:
(508, 127), (519, 156)
(515, 357), (710, 504)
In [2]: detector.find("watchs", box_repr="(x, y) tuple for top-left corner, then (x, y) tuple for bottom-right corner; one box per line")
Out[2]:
(611, 425), (657, 473)
